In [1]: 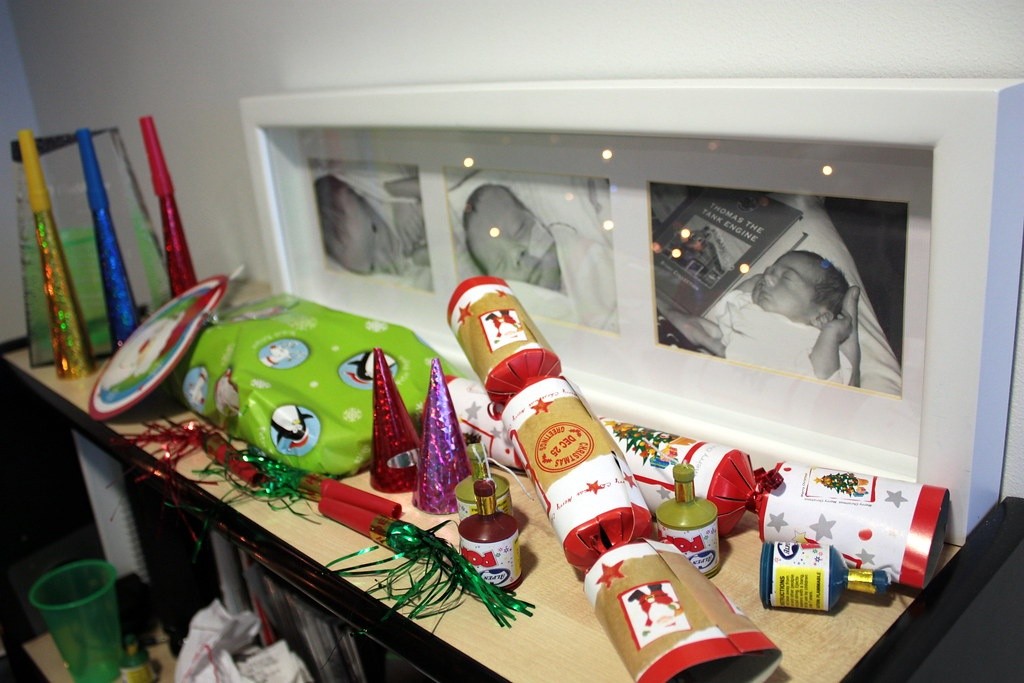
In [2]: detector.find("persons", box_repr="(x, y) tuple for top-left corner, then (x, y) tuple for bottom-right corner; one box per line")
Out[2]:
(656, 250), (861, 387)
(316, 172), (431, 275)
(463, 185), (618, 332)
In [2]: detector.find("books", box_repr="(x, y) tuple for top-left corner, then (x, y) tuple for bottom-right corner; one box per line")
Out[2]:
(656, 189), (808, 318)
(239, 550), (368, 683)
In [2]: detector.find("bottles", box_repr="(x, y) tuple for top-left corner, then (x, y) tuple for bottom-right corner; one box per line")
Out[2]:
(656, 464), (721, 578)
(455, 443), (513, 523)
(759, 540), (889, 611)
(459, 480), (522, 589)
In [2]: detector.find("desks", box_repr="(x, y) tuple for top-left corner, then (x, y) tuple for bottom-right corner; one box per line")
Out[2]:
(0, 315), (962, 683)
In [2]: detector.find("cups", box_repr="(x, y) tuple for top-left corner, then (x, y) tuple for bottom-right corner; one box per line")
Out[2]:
(28, 559), (122, 683)
